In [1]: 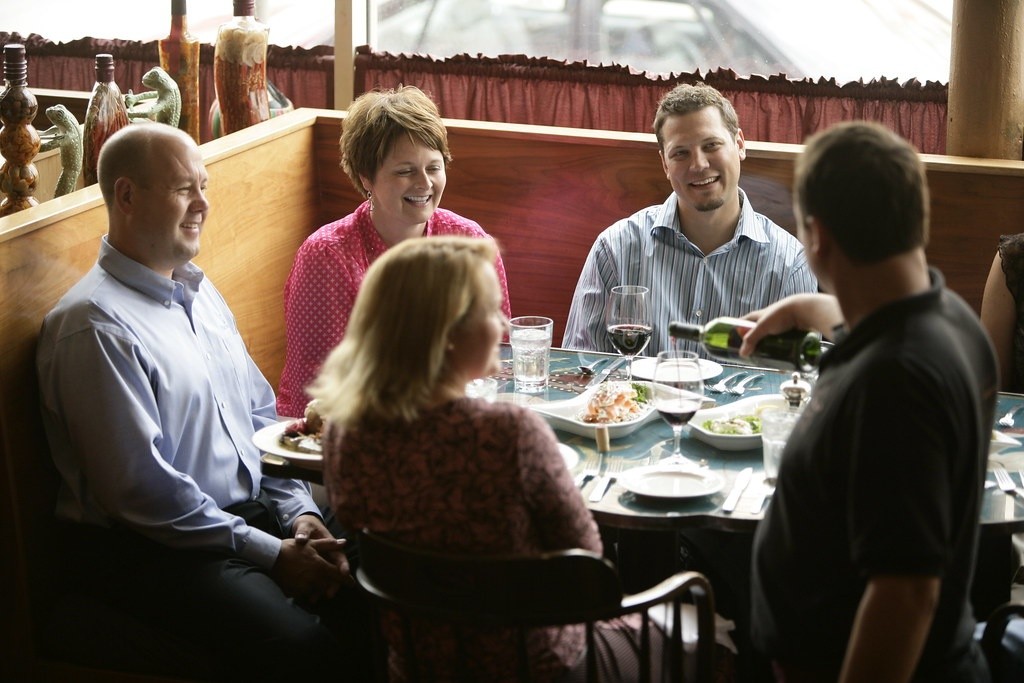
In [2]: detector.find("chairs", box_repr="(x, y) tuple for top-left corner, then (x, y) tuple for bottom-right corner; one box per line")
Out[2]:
(345, 504), (719, 683)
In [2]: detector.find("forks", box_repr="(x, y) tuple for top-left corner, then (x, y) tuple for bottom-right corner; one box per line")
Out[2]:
(994, 401), (1024, 426)
(573, 454), (602, 487)
(723, 373), (765, 394)
(993, 466), (1024, 500)
(703, 370), (748, 393)
(588, 455), (624, 503)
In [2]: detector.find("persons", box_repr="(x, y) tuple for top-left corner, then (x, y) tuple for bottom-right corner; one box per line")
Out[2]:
(314, 237), (728, 681)
(39, 122), (379, 682)
(561, 80), (820, 364)
(278, 85), (516, 416)
(739, 121), (998, 683)
(982, 235), (1024, 392)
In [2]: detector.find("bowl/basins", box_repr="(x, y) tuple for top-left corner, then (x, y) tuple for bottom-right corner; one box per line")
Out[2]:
(523, 381), (653, 440)
(688, 393), (785, 451)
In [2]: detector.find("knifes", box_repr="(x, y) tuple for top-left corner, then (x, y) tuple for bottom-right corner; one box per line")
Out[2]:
(584, 358), (625, 389)
(722, 467), (753, 511)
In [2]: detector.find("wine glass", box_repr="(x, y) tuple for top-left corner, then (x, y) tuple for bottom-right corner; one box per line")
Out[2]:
(653, 349), (704, 467)
(604, 285), (653, 381)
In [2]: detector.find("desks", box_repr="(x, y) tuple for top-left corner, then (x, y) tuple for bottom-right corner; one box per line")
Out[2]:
(254, 342), (1024, 683)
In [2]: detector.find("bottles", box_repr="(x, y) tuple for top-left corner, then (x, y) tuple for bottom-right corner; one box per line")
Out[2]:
(760, 373), (813, 482)
(0, 44), (41, 218)
(158, 0), (200, 146)
(670, 315), (823, 373)
(215, 0), (272, 134)
(83, 52), (130, 185)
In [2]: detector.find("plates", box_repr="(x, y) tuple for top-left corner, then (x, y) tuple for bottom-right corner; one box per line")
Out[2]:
(557, 441), (579, 474)
(617, 465), (725, 499)
(252, 419), (323, 470)
(626, 357), (723, 381)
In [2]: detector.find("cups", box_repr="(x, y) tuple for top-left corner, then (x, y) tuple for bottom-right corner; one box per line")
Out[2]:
(508, 315), (554, 393)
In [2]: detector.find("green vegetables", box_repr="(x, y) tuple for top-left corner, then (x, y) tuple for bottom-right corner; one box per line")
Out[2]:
(630, 382), (651, 404)
(704, 414), (761, 435)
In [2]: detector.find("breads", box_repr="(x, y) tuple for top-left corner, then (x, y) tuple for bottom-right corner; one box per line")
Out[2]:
(583, 382), (639, 424)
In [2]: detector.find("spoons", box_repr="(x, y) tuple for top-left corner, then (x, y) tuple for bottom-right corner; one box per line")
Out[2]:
(579, 358), (608, 372)
